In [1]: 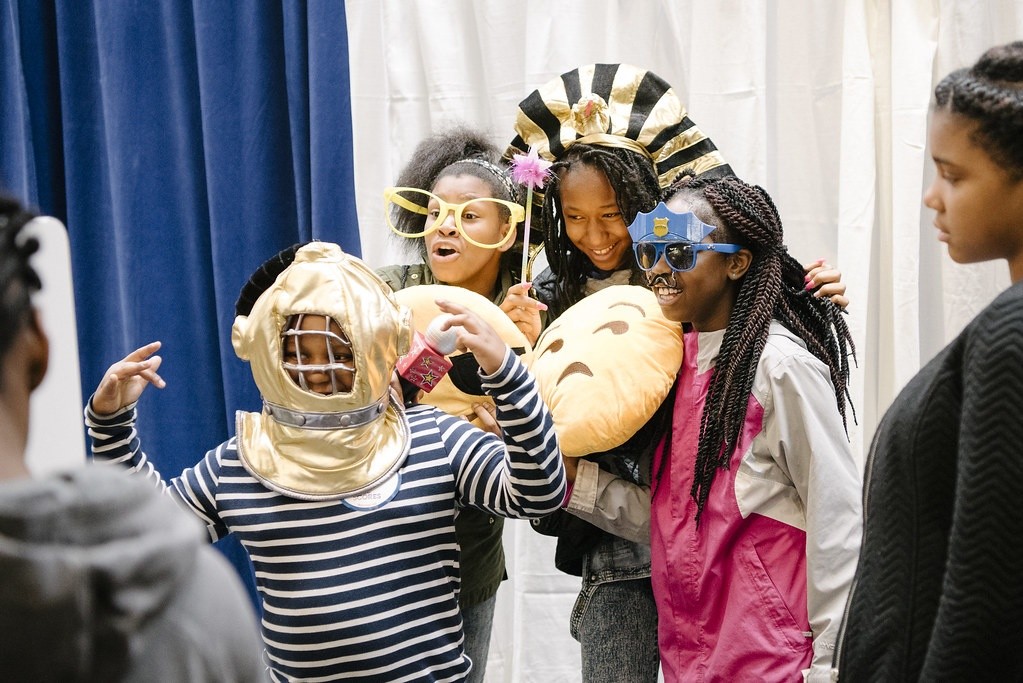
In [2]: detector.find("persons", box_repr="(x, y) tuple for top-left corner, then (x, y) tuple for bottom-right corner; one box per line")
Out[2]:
(84, 242), (568, 682)
(830, 39), (1023, 683)
(372, 63), (861, 682)
(0, 196), (266, 682)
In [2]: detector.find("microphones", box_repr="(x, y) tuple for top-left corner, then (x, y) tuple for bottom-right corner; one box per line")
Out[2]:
(395, 312), (464, 405)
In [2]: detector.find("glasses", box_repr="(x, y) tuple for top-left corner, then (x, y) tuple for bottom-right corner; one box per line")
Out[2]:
(627, 202), (742, 271)
(383, 187), (525, 248)
